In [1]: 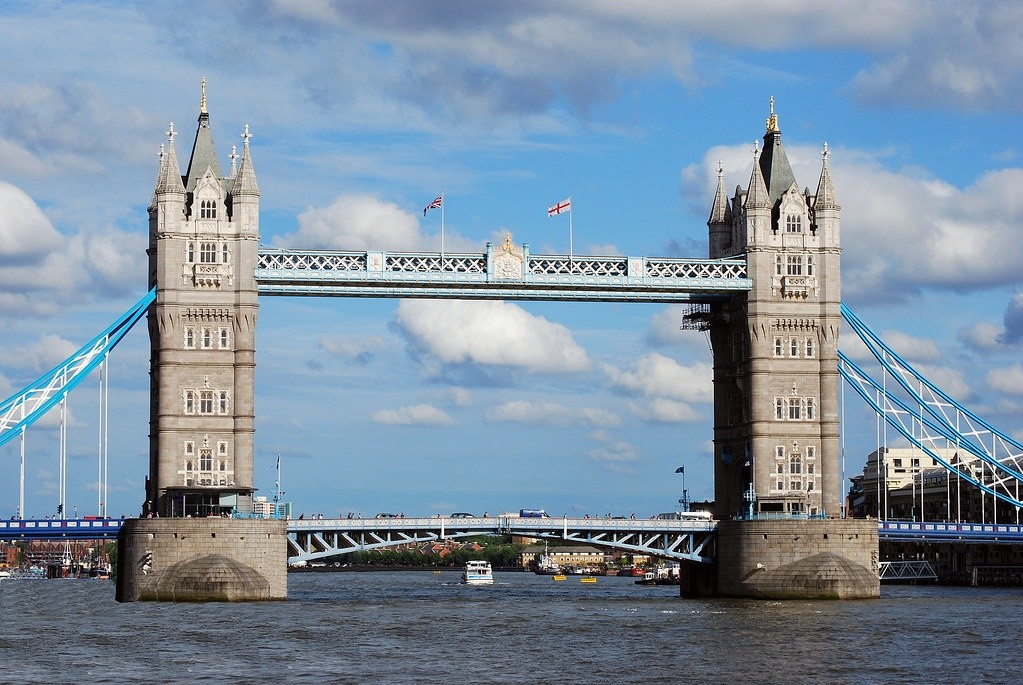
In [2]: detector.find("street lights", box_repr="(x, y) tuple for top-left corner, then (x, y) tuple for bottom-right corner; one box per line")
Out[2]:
(683, 490), (687, 511)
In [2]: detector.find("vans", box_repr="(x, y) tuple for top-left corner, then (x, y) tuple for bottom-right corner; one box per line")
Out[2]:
(656, 513), (679, 519)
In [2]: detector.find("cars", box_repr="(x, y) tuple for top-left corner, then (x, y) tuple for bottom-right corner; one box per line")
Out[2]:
(375, 514), (396, 517)
(450, 512), (475, 517)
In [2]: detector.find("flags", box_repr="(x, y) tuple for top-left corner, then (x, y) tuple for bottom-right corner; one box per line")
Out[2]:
(422, 194), (442, 217)
(675, 467), (684, 473)
(276, 453), (279, 469)
(547, 198), (571, 218)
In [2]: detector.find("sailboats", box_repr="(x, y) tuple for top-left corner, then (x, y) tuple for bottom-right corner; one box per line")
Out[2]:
(534, 539), (565, 575)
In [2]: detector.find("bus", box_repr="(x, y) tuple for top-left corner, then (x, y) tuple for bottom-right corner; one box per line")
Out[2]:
(680, 512), (713, 521)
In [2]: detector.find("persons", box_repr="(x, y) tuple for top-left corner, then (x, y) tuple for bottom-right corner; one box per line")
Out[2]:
(484, 511), (489, 518)
(120, 511), (159, 520)
(187, 511), (230, 518)
(437, 513), (439, 518)
(286, 512), (405, 520)
(10, 515), (79, 520)
(728, 511), (746, 520)
(563, 513), (657, 519)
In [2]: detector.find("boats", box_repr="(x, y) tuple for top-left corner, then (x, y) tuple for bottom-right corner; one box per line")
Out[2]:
(581, 576), (596, 582)
(460, 561), (495, 585)
(548, 567), (562, 575)
(582, 563), (617, 576)
(89, 567), (110, 579)
(616, 566), (645, 577)
(552, 574), (566, 581)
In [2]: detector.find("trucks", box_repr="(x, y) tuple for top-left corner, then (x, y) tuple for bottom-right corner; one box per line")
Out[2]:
(520, 509), (550, 518)
